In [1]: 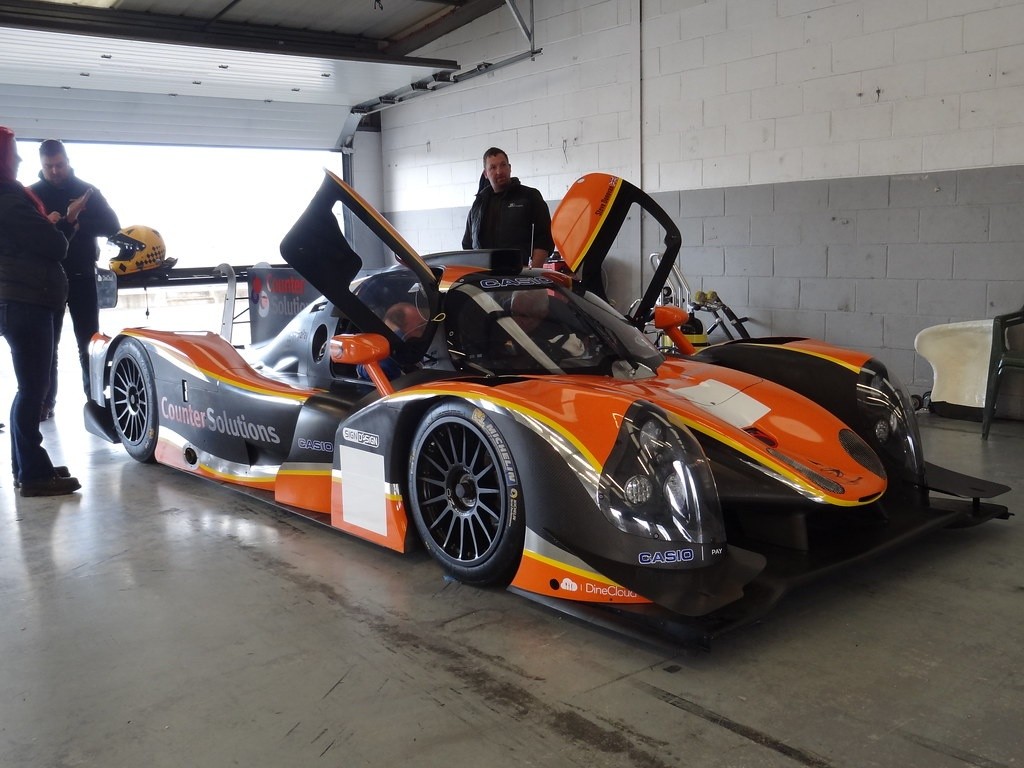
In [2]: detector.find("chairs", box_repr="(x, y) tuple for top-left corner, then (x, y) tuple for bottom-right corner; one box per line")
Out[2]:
(383, 302), (429, 341)
(510, 289), (548, 335)
(982, 305), (1024, 441)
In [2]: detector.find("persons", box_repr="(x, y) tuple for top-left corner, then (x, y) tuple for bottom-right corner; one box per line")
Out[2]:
(462, 147), (555, 268)
(0, 126), (121, 498)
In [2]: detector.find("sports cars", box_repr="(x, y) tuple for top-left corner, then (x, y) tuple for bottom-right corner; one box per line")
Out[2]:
(83, 168), (1015, 655)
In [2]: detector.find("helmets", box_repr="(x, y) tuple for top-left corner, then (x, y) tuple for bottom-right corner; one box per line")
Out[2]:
(106, 225), (167, 276)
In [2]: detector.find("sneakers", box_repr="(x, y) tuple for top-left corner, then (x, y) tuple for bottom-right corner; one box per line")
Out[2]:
(40, 401), (56, 421)
(12, 466), (82, 497)
